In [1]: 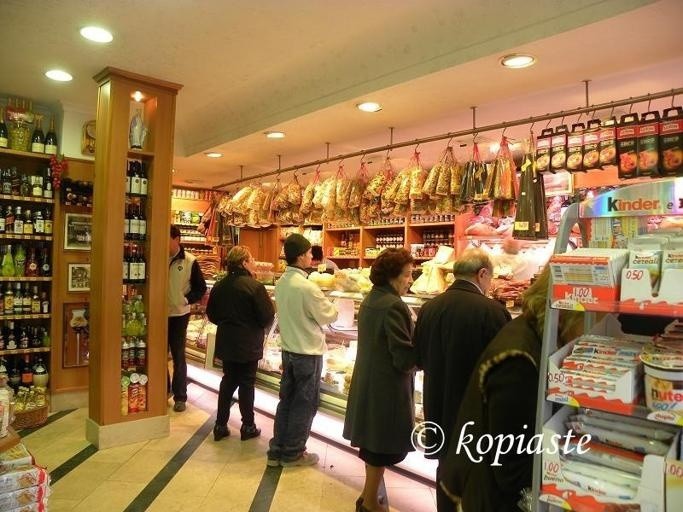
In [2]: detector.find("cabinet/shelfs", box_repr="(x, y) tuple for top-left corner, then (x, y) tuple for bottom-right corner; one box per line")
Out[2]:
(91, 68), (182, 452)
(0, 94), (56, 393)
(533, 178), (683, 512)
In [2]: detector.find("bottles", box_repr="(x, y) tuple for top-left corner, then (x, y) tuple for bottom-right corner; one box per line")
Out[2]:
(339, 232), (354, 251)
(182, 245), (195, 254)
(119, 156), (147, 373)
(0, 96), (57, 393)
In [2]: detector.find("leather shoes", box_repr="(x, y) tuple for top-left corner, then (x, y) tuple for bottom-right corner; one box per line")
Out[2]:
(356, 495), (384, 512)
(214, 424), (230, 441)
(174, 401), (185, 412)
(241, 424), (261, 440)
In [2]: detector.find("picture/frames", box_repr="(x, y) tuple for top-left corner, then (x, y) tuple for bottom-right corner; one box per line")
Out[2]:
(61, 212), (92, 369)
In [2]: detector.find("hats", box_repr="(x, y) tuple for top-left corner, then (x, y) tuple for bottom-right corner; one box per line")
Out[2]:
(284, 234), (312, 257)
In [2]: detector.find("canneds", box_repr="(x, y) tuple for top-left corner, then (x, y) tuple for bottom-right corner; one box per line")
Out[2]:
(416, 230), (454, 256)
(177, 226), (207, 242)
(375, 234), (403, 250)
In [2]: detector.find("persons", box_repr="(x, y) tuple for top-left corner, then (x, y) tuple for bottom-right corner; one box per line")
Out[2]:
(204, 245), (275, 441)
(261, 231), (341, 473)
(440, 251), (587, 512)
(167, 223), (208, 414)
(343, 246), (417, 512)
(411, 246), (512, 512)
(309, 244), (340, 273)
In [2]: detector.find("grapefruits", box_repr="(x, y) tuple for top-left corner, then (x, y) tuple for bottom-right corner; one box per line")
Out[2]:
(307, 271), (335, 287)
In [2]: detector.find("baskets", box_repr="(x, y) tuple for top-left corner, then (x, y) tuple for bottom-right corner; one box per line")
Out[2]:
(11, 391), (49, 429)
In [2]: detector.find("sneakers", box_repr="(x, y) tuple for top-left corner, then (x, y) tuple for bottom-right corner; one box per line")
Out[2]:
(267, 459), (279, 466)
(281, 452), (319, 467)
